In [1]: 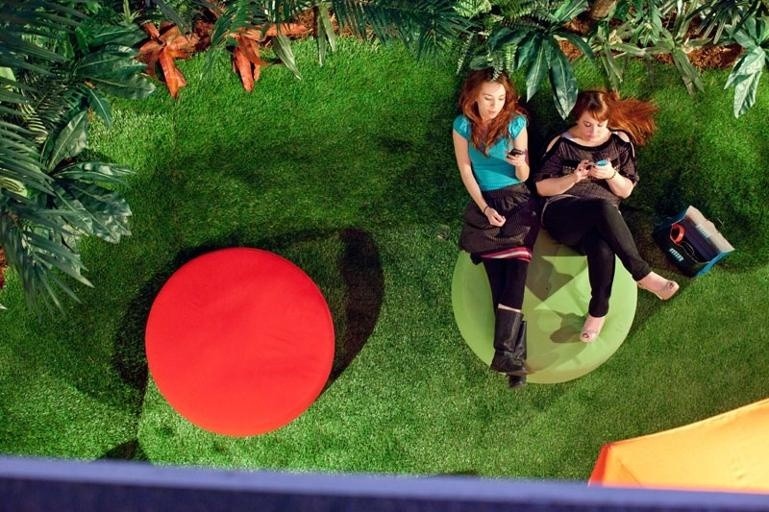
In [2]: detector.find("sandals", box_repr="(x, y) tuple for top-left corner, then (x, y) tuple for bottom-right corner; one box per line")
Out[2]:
(638, 278), (680, 301)
(580, 315), (604, 342)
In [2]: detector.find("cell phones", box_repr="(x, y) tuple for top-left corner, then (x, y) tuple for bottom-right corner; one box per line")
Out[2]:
(507, 149), (522, 160)
(595, 159), (609, 166)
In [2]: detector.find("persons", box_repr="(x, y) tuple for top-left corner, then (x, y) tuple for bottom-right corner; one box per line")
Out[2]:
(536, 88), (681, 344)
(452, 67), (540, 390)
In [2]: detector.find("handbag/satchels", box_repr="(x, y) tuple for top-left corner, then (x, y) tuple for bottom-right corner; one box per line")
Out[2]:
(653, 205), (737, 276)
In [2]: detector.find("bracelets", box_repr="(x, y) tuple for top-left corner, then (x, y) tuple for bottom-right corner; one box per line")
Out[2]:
(604, 167), (617, 183)
(481, 203), (489, 217)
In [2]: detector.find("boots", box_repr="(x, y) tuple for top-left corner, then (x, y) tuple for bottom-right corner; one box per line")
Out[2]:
(490, 308), (529, 388)
